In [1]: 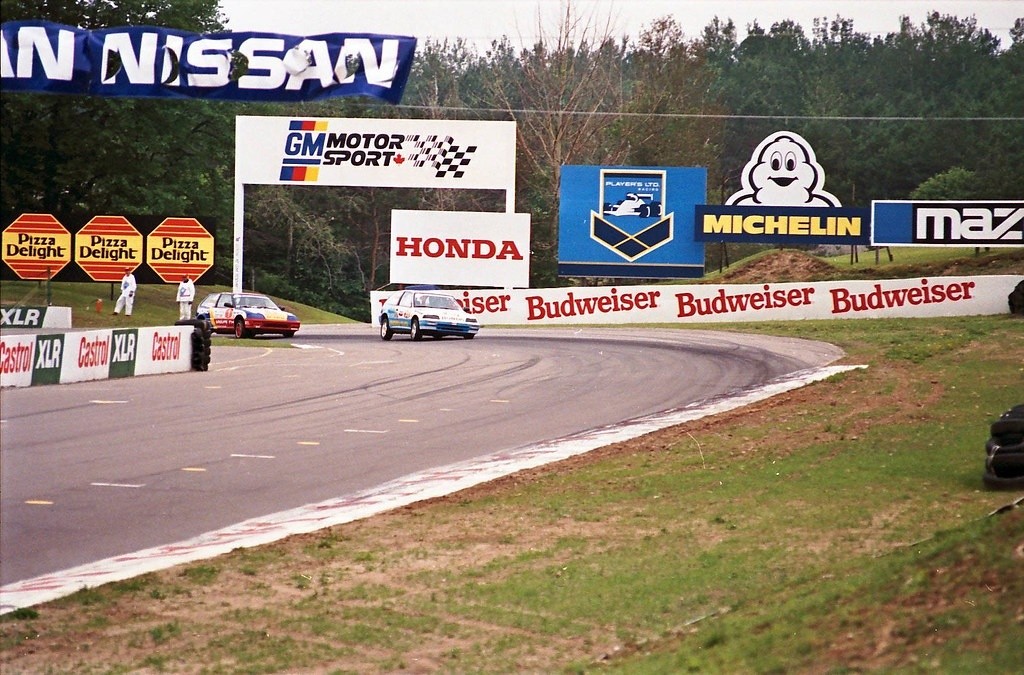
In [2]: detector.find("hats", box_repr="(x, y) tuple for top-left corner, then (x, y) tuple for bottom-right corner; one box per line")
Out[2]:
(182, 274), (189, 279)
(124, 267), (131, 270)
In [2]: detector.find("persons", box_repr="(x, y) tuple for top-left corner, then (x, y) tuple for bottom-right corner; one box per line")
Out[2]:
(111, 267), (137, 317)
(176, 275), (196, 321)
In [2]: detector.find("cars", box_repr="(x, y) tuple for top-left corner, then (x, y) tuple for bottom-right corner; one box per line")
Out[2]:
(377, 289), (479, 343)
(196, 290), (301, 340)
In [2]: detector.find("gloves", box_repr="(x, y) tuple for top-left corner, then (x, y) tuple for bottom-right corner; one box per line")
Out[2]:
(129, 294), (132, 297)
(188, 301), (193, 305)
